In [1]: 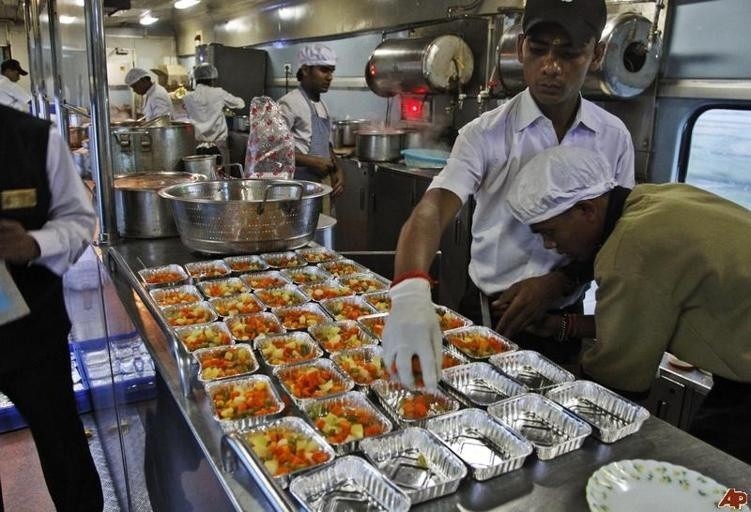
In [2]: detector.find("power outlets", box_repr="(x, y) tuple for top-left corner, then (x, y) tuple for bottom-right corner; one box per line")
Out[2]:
(283, 63), (294, 73)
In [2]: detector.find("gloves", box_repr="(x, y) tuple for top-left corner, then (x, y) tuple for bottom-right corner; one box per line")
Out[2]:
(380, 272), (446, 397)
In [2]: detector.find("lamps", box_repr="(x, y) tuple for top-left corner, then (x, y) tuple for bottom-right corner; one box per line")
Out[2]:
(140, 9), (162, 28)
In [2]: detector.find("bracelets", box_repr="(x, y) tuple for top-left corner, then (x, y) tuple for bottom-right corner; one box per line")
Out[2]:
(568, 313), (578, 338)
(559, 312), (568, 346)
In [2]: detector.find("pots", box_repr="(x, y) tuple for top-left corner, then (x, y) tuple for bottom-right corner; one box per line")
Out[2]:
(161, 162), (335, 256)
(107, 121), (195, 173)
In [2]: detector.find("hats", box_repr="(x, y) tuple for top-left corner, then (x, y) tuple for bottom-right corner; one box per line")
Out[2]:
(125, 67), (152, 87)
(294, 41), (339, 76)
(192, 62), (219, 82)
(1, 58), (29, 76)
(520, 0), (608, 48)
(500, 144), (618, 226)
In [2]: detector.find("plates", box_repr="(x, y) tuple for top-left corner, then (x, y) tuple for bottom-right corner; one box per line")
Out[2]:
(587, 456), (749, 512)
(136, 247), (649, 510)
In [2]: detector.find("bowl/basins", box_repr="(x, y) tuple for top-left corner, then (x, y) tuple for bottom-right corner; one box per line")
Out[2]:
(233, 116), (250, 133)
(184, 155), (219, 178)
(336, 119), (451, 171)
(113, 172), (200, 239)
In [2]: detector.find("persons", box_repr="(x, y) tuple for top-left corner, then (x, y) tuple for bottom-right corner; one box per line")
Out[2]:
(0, 102), (99, 511)
(382, 0), (638, 395)
(182, 61), (246, 178)
(275, 43), (345, 218)
(0, 59), (32, 113)
(494, 147), (751, 464)
(125, 67), (175, 122)
